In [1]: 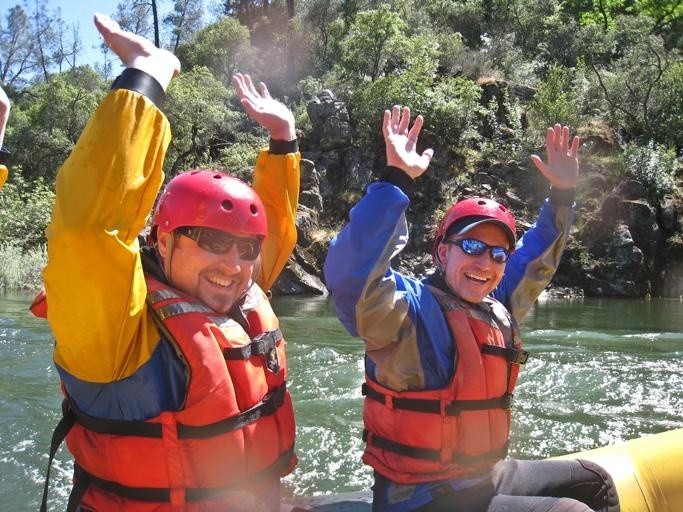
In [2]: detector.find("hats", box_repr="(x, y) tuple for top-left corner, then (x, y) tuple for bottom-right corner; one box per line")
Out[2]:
(456, 213), (516, 248)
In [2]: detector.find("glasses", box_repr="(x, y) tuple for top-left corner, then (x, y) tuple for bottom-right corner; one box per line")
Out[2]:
(173, 226), (264, 260)
(442, 237), (512, 265)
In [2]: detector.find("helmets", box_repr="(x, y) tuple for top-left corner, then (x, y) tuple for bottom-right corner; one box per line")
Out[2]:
(431, 196), (517, 265)
(145, 168), (270, 256)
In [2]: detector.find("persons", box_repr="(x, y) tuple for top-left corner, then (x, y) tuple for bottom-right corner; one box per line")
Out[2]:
(0, 82), (14, 193)
(26, 10), (302, 512)
(317, 100), (623, 512)
